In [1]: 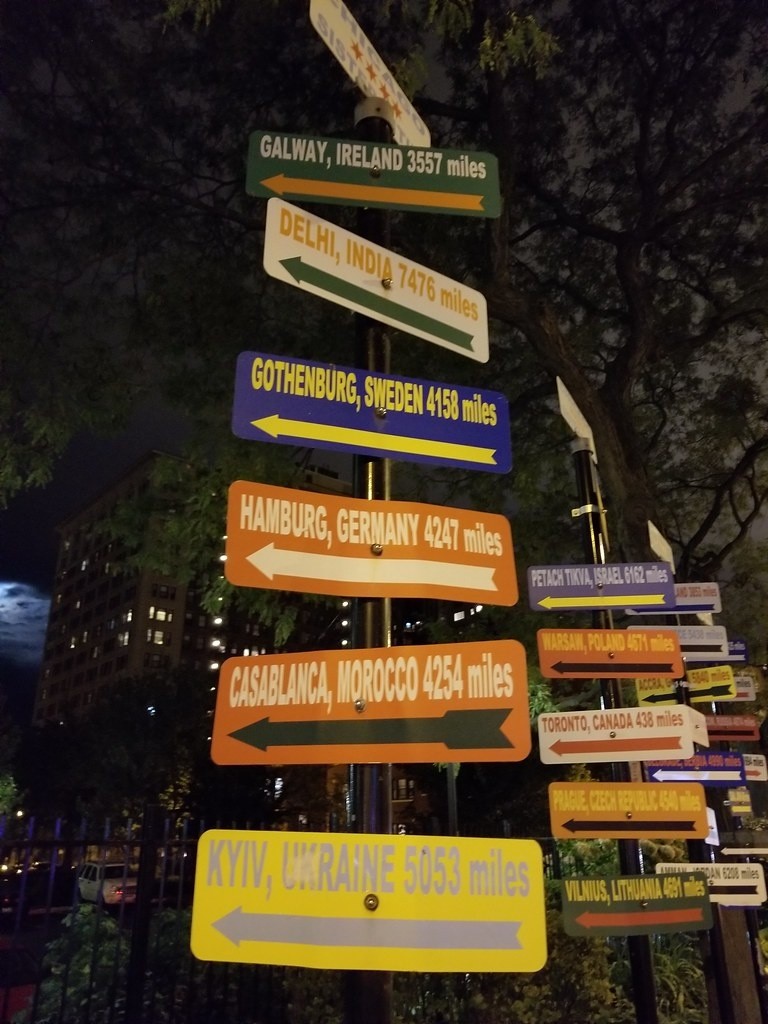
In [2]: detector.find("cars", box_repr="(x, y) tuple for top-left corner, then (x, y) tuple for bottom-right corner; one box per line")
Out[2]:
(78, 861), (137, 903)
(0, 862), (70, 903)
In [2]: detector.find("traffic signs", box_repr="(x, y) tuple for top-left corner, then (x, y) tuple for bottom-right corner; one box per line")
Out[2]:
(647, 520), (675, 574)
(231, 350), (513, 475)
(704, 808), (720, 846)
(561, 872), (715, 938)
(556, 375), (597, 464)
(728, 789), (752, 816)
(706, 715), (760, 741)
(695, 613), (713, 625)
(528, 561), (677, 610)
(310, 0), (431, 147)
(743, 753), (768, 782)
(627, 626), (729, 661)
(726, 640), (748, 662)
(647, 751), (746, 786)
(625, 583), (722, 615)
(654, 862), (767, 905)
(263, 196), (490, 364)
(538, 628), (684, 678)
(190, 828), (548, 973)
(224, 478), (520, 606)
(635, 665), (737, 707)
(538, 703), (696, 764)
(734, 677), (756, 702)
(245, 129), (502, 218)
(211, 638), (531, 766)
(548, 782), (709, 840)
